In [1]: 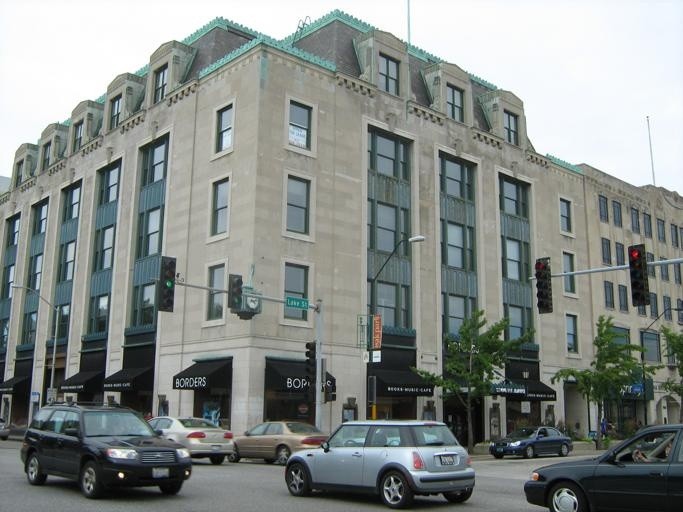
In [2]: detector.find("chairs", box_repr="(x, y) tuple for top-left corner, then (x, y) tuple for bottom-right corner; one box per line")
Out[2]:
(372, 433), (387, 447)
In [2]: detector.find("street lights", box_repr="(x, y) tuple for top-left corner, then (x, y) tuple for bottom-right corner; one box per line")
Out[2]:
(228, 274), (243, 309)
(12, 285), (61, 403)
(157, 256), (176, 312)
(371, 235), (426, 420)
(639, 308), (683, 426)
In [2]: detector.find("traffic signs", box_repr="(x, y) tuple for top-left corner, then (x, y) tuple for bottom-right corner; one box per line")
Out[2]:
(286, 296), (309, 310)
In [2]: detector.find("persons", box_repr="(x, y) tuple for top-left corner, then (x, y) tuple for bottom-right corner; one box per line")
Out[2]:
(600, 418), (607, 438)
(632, 440), (673, 464)
(210, 403), (222, 428)
(143, 412), (153, 421)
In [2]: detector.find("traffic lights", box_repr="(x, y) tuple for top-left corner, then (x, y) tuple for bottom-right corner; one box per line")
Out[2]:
(534, 257), (553, 314)
(628, 244), (651, 308)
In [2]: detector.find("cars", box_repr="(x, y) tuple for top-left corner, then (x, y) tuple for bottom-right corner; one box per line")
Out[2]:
(524, 424), (683, 512)
(489, 426), (574, 459)
